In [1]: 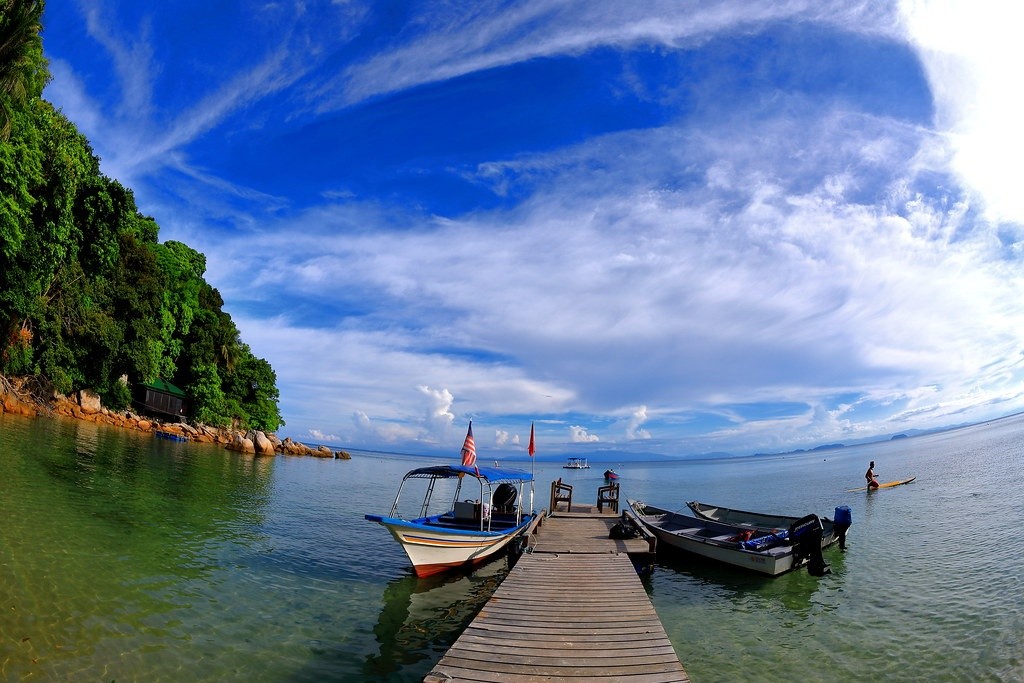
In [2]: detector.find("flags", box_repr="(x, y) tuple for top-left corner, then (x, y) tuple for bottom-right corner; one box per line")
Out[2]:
(526, 423), (536, 457)
(459, 421), (476, 477)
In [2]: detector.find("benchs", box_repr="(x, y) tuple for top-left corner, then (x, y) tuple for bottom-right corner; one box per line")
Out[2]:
(425, 516), (516, 532)
(597, 481), (620, 513)
(644, 514), (738, 541)
(550, 478), (573, 512)
(740, 520), (792, 530)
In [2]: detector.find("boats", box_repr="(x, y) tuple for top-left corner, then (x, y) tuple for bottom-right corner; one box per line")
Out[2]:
(365, 417), (538, 579)
(685, 501), (852, 549)
(627, 499), (832, 577)
(563, 457), (591, 469)
(603, 469), (618, 480)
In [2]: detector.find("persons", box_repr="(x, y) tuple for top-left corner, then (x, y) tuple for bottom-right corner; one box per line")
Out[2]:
(865, 461), (879, 493)
